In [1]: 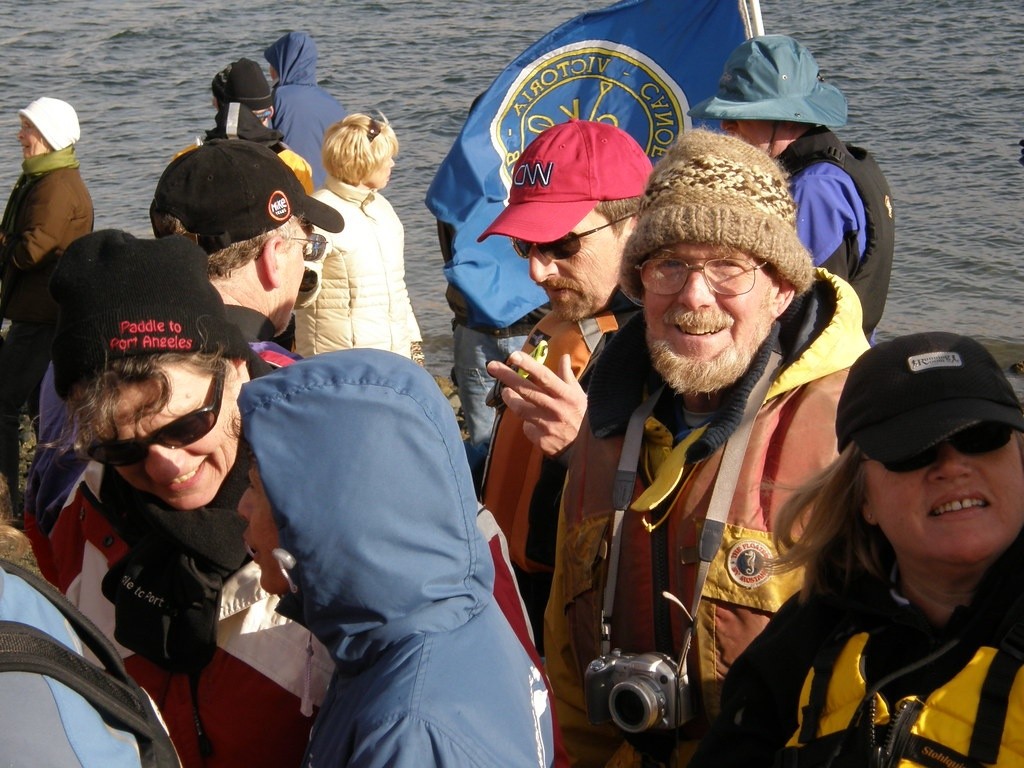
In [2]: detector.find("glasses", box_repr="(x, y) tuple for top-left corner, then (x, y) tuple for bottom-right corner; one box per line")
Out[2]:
(509, 212), (638, 260)
(254, 232), (328, 261)
(860, 421), (1013, 470)
(367, 118), (381, 143)
(635, 254), (767, 296)
(86, 356), (227, 468)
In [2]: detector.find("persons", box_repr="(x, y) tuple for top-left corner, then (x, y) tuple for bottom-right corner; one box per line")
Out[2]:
(236, 348), (554, 768)
(483, 120), (654, 620)
(150, 138), (344, 367)
(543, 129), (872, 768)
(294, 113), (426, 367)
(21, 230), (570, 768)
(687, 34), (896, 340)
(434, 220), (554, 470)
(174, 58), (315, 196)
(0, 97), (95, 518)
(688, 330), (1024, 768)
(264, 32), (346, 194)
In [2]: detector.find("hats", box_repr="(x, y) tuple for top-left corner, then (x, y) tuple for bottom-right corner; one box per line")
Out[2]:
(477, 118), (655, 243)
(18, 96), (81, 151)
(149, 138), (345, 252)
(687, 34), (848, 127)
(618, 130), (813, 305)
(836, 331), (1024, 462)
(50, 228), (249, 404)
(212, 57), (274, 111)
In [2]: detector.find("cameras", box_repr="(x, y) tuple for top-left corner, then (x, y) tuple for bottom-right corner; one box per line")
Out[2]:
(584, 648), (694, 733)
